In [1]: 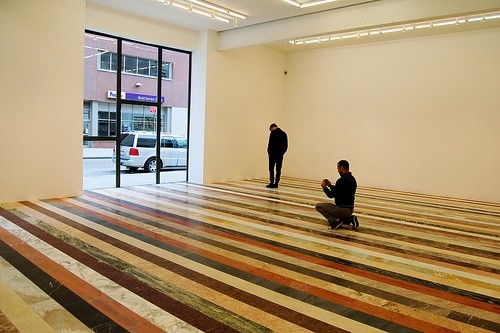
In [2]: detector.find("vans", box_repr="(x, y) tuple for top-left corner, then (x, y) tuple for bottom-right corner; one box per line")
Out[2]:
(112, 131), (187, 173)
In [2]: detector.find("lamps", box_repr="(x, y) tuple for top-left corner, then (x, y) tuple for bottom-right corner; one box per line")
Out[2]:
(289, 10), (500, 47)
(161, 0), (247, 23)
(136, 83), (142, 85)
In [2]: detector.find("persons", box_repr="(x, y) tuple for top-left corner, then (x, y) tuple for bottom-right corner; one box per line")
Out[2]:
(316, 160), (359, 230)
(266, 124), (288, 188)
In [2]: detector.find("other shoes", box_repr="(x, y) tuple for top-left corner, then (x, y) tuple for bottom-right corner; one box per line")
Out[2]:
(329, 220), (343, 230)
(352, 215), (359, 228)
(266, 183), (278, 188)
